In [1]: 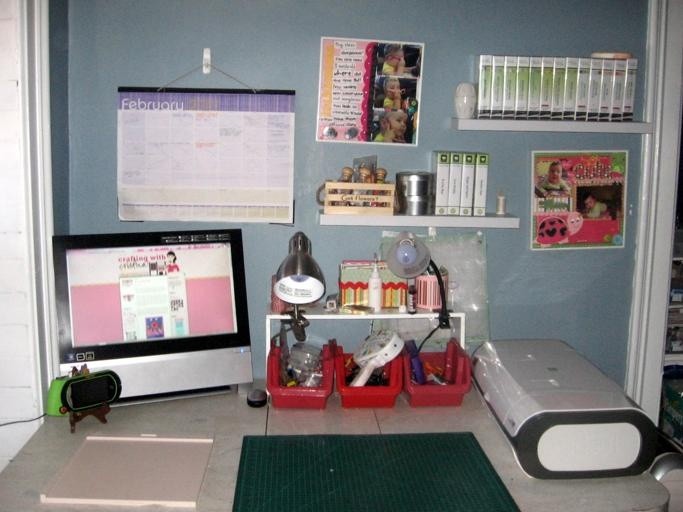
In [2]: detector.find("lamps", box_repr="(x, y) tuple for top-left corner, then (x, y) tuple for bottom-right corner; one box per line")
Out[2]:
(386, 231), (453, 329)
(274, 232), (326, 342)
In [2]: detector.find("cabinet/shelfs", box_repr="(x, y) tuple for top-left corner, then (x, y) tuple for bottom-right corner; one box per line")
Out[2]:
(264, 308), (465, 380)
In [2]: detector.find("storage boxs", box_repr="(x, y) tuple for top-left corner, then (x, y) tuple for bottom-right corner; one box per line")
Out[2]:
(267, 343), (333, 409)
(407, 351), (472, 406)
(335, 342), (404, 407)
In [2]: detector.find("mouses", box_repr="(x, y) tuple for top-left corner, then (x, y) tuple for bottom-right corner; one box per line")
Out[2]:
(245, 386), (267, 408)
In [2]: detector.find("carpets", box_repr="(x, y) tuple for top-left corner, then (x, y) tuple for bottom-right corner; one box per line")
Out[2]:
(231, 431), (520, 512)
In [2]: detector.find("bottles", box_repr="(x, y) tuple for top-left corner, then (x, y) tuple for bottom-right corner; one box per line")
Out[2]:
(368, 262), (383, 313)
(331, 166), (386, 206)
(496, 196), (507, 215)
(407, 284), (417, 314)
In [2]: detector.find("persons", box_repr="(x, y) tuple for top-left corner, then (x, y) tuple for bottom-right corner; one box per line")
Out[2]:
(381, 44), (412, 76)
(370, 110), (408, 144)
(583, 195), (608, 219)
(380, 76), (407, 110)
(166, 252), (179, 272)
(536, 160), (570, 197)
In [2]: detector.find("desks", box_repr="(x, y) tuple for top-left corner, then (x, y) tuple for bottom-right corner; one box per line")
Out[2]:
(0, 379), (670, 511)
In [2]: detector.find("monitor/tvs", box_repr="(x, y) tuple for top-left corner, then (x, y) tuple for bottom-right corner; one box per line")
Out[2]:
(50, 226), (256, 408)
